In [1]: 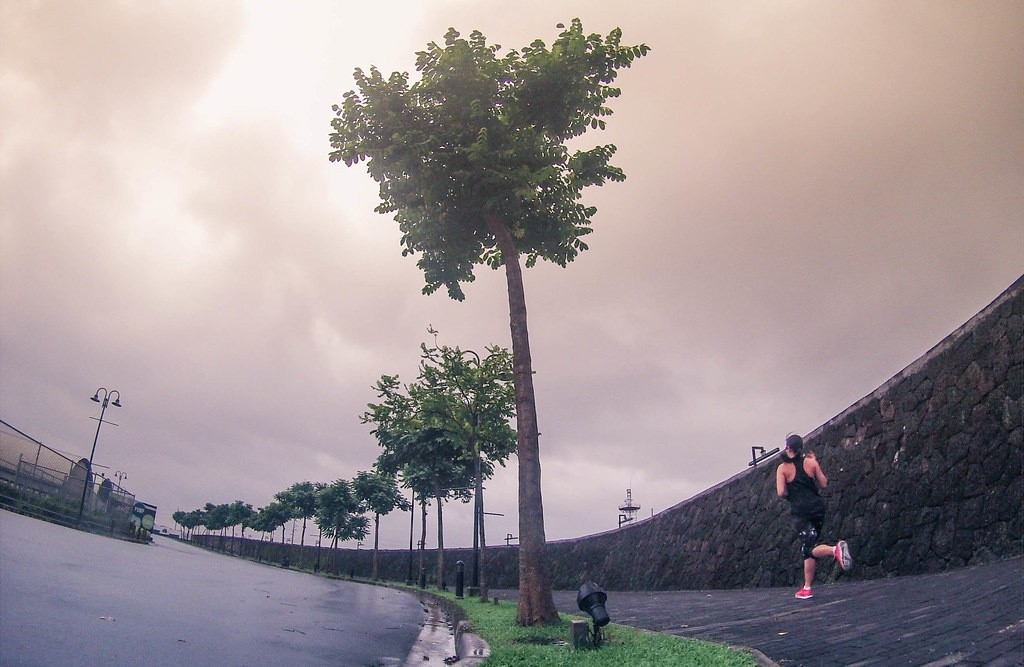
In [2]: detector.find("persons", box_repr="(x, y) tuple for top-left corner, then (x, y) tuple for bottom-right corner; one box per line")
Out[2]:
(776, 433), (852, 599)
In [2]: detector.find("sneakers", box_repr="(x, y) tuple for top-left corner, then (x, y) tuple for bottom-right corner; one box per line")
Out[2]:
(835, 541), (852, 570)
(795, 587), (813, 599)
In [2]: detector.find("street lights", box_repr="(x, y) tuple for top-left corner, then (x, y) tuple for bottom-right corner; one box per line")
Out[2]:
(114, 470), (127, 494)
(452, 350), (514, 602)
(74, 386), (122, 529)
